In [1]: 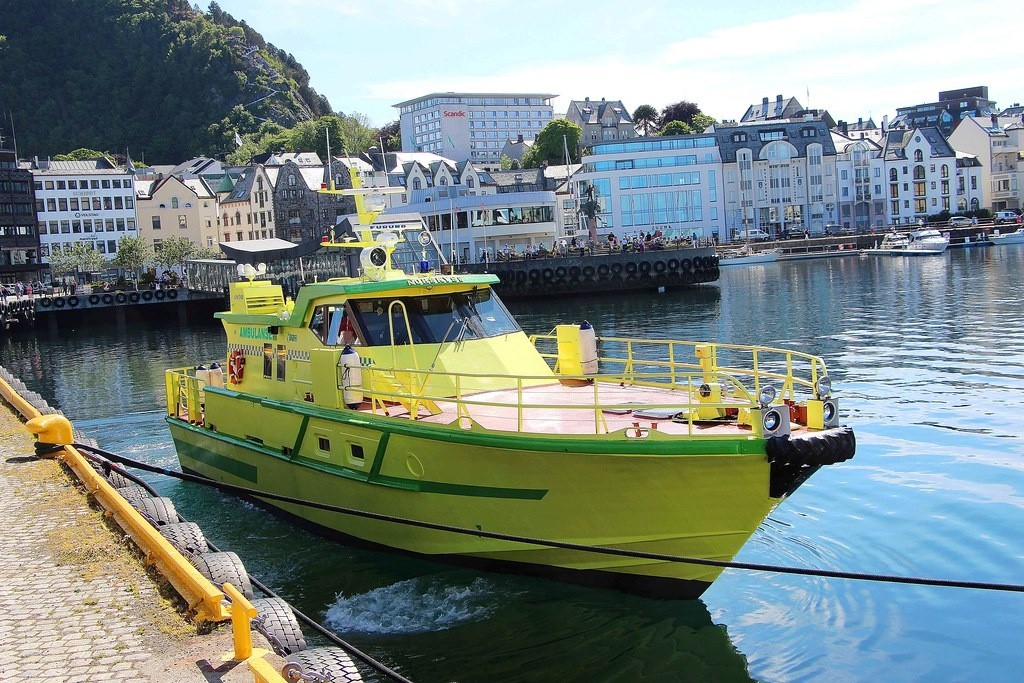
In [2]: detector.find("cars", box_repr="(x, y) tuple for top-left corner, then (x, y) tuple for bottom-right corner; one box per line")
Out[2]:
(1, 284), (16, 296)
(780, 228), (806, 240)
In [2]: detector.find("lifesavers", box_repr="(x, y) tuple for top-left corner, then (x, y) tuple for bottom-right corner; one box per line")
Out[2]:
(0, 363), (365, 683)
(0, 289), (179, 317)
(490, 254), (721, 295)
(228, 350), (244, 385)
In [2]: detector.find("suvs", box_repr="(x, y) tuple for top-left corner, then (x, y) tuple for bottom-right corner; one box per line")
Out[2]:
(948, 217), (972, 228)
(825, 225), (843, 236)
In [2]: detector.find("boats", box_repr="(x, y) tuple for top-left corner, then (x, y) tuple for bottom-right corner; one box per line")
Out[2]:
(879, 226), (911, 249)
(989, 229), (1024, 245)
(164, 128), (855, 604)
(907, 227), (949, 252)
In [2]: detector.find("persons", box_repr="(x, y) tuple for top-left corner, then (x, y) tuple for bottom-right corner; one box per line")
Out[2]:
(803, 228), (810, 240)
(972, 215), (978, 225)
(993, 215), (999, 224)
(1013, 212), (1024, 224)
(870, 224), (874, 235)
(919, 218), (926, 228)
(0, 277), (85, 302)
(95, 272), (188, 293)
(823, 226), (854, 236)
(891, 226), (897, 235)
(451, 229), (702, 265)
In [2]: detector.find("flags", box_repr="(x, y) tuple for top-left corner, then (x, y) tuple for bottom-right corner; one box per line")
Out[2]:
(235, 132), (244, 147)
(454, 203), (459, 213)
(481, 201), (485, 211)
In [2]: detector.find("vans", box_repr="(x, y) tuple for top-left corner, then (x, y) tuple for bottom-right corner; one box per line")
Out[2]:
(995, 211), (1017, 224)
(748, 230), (770, 241)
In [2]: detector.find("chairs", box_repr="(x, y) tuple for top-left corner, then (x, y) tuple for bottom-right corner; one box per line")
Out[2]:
(366, 312), (388, 325)
